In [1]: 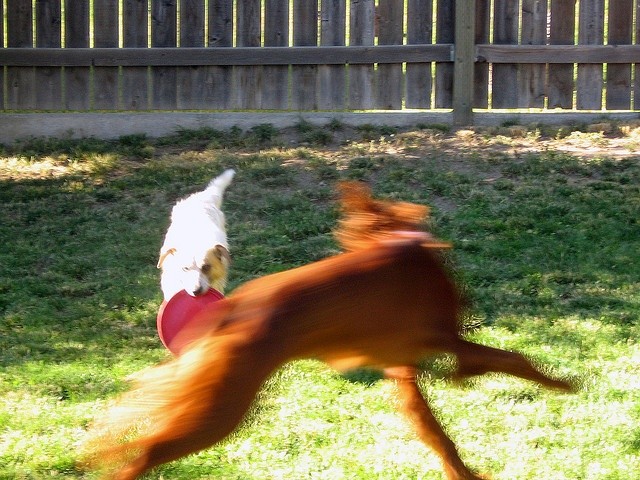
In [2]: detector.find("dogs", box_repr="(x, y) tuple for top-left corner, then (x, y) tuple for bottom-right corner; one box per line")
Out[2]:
(158, 169), (235, 303)
(97, 177), (572, 476)
